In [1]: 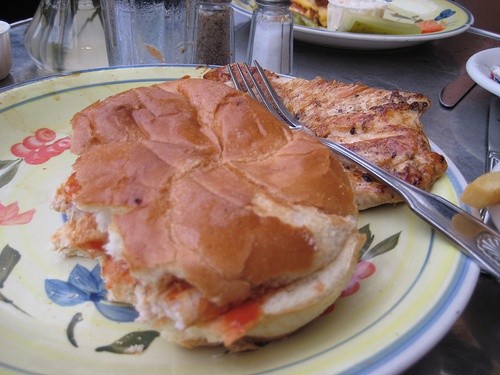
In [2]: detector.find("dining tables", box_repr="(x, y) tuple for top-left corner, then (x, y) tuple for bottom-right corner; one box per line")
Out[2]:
(290, 25), (500, 375)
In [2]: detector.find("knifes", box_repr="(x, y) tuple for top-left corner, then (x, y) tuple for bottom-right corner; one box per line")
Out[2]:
(440, 68), (477, 108)
(480, 94), (500, 227)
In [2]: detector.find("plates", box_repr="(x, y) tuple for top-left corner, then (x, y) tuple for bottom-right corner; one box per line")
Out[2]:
(0, 65), (479, 375)
(465, 48), (500, 97)
(231, 0), (475, 48)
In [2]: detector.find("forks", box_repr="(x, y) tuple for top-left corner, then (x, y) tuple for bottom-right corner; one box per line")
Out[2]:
(228, 59), (500, 280)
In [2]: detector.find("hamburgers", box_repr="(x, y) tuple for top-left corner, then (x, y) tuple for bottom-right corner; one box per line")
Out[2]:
(51, 78), (367, 352)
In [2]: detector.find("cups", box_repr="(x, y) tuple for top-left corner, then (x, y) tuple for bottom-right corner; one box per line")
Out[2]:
(100, 0), (195, 66)
(0, 21), (12, 82)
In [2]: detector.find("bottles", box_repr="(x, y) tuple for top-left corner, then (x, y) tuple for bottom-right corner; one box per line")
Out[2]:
(194, 0), (236, 68)
(246, 0), (294, 76)
